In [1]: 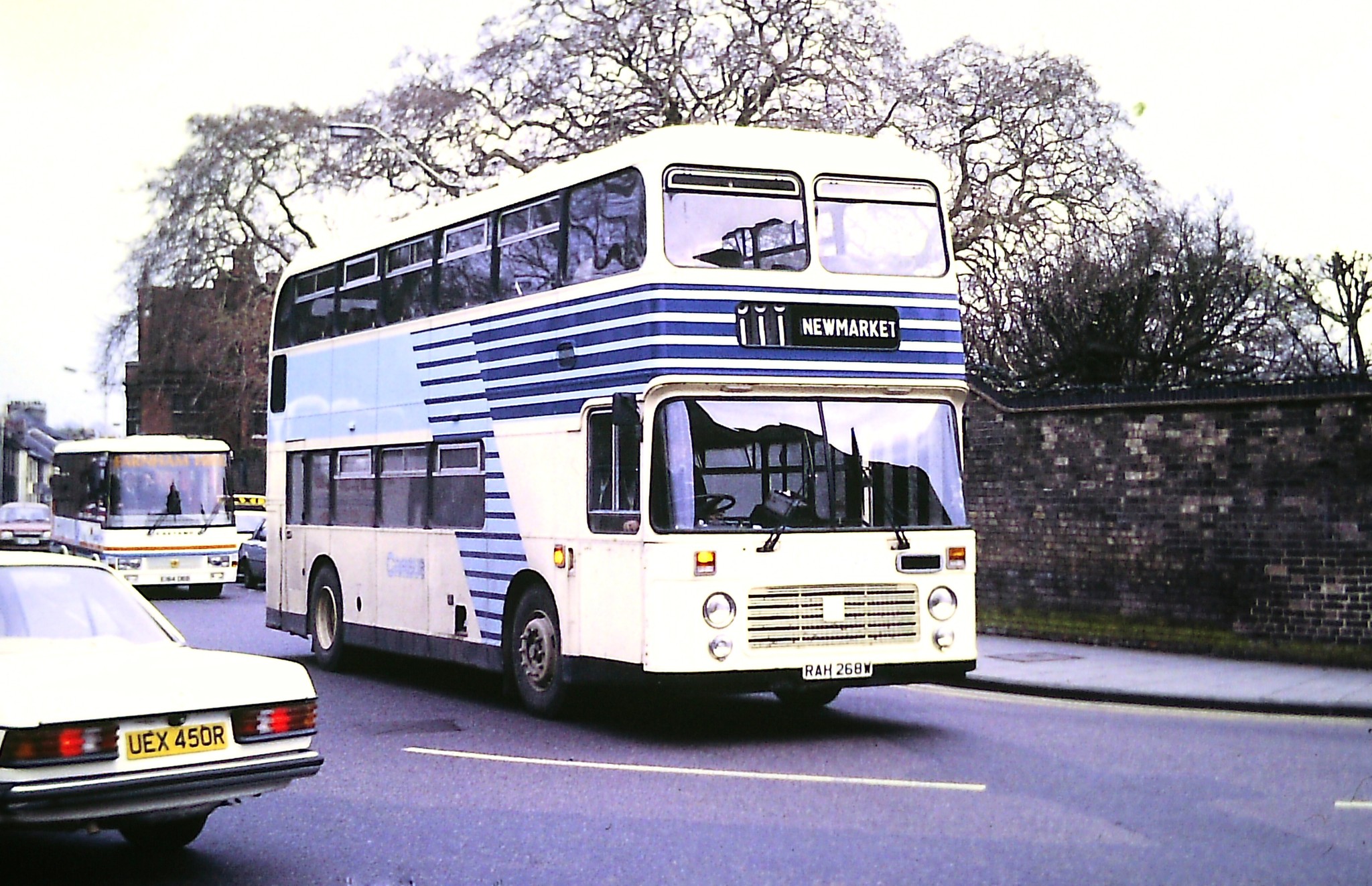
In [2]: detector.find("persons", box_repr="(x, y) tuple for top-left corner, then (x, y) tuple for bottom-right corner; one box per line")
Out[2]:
(623, 462), (710, 532)
(121, 474), (143, 510)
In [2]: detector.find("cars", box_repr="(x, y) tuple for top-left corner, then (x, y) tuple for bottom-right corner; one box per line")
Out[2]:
(0, 502), (54, 552)
(232, 495), (267, 546)
(0, 551), (326, 857)
(238, 521), (267, 589)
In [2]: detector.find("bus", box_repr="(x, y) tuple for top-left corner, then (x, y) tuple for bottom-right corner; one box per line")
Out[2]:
(45, 435), (239, 596)
(267, 122), (984, 708)
